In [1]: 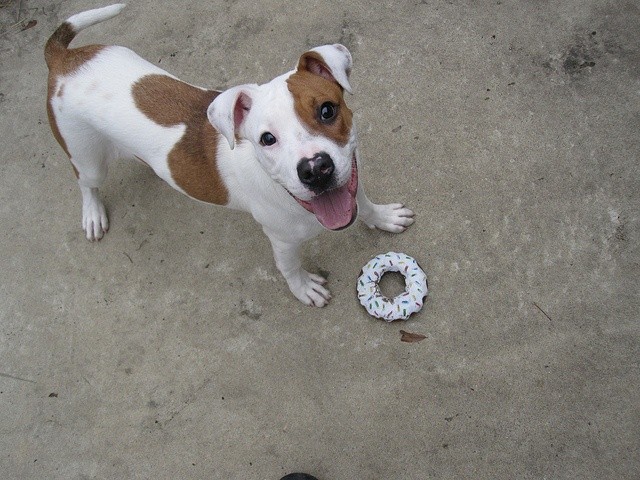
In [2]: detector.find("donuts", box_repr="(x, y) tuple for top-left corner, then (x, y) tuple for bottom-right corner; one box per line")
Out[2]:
(355, 250), (430, 323)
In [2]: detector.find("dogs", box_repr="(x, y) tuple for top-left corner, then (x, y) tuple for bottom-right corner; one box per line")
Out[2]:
(42, 2), (416, 309)
